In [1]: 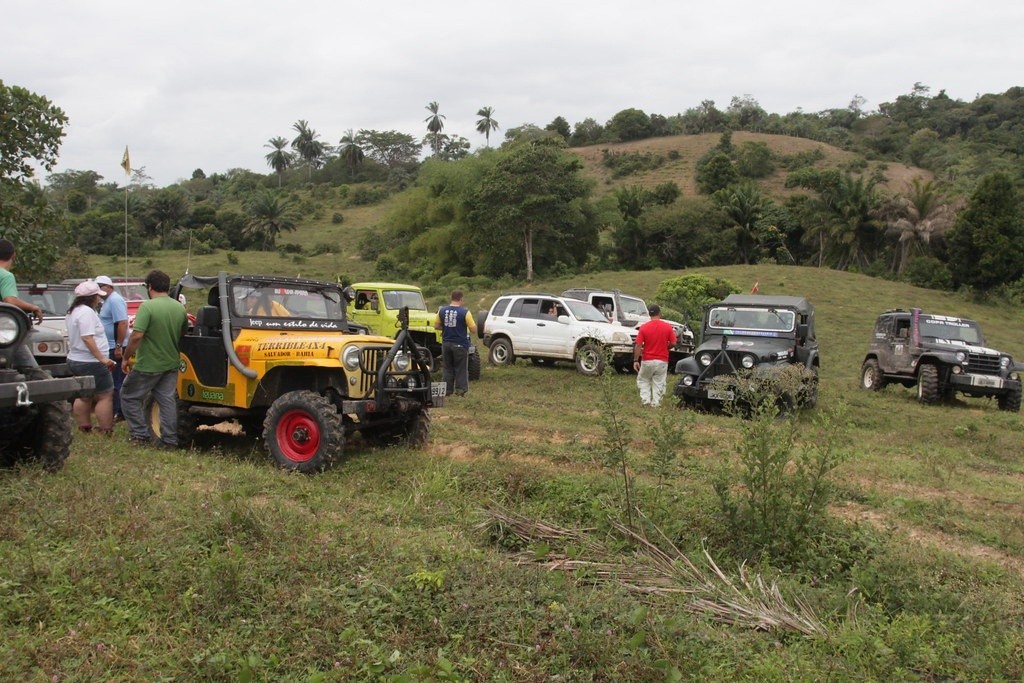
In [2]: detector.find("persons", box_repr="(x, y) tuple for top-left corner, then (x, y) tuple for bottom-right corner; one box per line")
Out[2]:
(175, 293), (197, 327)
(633, 304), (677, 411)
(362, 292), (380, 311)
(595, 298), (611, 314)
(244, 285), (294, 318)
(119, 271), (190, 451)
(434, 289), (478, 397)
(94, 274), (132, 424)
(0, 238), (43, 375)
(63, 280), (117, 442)
(542, 301), (558, 318)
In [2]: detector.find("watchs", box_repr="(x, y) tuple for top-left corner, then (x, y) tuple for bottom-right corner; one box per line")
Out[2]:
(116, 343), (123, 349)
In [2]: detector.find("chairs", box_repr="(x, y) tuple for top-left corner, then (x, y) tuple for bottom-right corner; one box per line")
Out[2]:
(192, 286), (238, 335)
(763, 313), (789, 330)
(355, 292), (371, 310)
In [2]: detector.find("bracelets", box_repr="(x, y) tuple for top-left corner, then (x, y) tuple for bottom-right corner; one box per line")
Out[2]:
(633, 360), (639, 362)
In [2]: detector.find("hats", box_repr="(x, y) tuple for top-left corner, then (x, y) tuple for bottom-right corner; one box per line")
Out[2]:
(649, 304), (660, 313)
(237, 288), (262, 299)
(95, 275), (112, 286)
(75, 281), (107, 297)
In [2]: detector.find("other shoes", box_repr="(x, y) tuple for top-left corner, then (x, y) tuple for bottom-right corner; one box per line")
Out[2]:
(129, 437), (150, 446)
(155, 441), (177, 450)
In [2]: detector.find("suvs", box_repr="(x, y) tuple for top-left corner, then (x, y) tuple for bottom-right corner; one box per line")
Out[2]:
(532, 288), (695, 374)
(61, 277), (196, 333)
(859, 308), (1024, 412)
(674, 293), (820, 418)
(126, 270), (434, 474)
(344, 282), (480, 381)
(477, 292), (644, 377)
(15, 283), (78, 379)
(1, 301), (96, 477)
